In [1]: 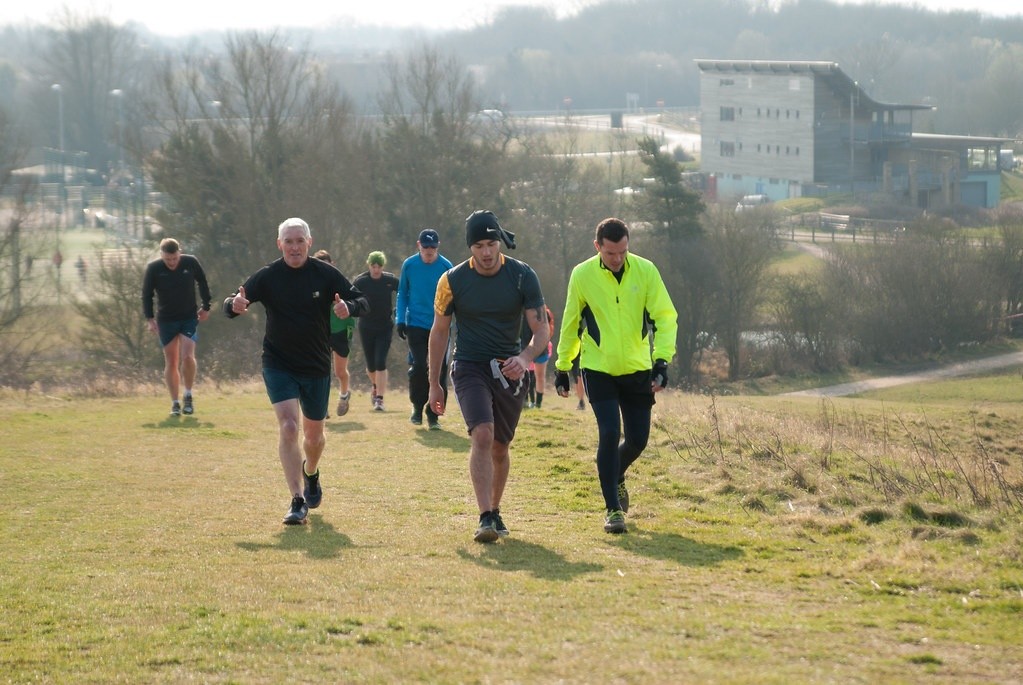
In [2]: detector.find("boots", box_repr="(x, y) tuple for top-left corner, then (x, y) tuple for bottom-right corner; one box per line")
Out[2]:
(535, 391), (543, 408)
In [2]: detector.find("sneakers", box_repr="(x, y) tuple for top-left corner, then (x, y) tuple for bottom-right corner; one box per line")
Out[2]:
(183, 395), (193, 414)
(337, 391), (351, 416)
(493, 509), (509, 536)
(617, 483), (629, 513)
(371, 396), (383, 409)
(281, 499), (308, 524)
(302, 460), (322, 508)
(474, 512), (498, 541)
(604, 510), (627, 533)
(171, 401), (181, 416)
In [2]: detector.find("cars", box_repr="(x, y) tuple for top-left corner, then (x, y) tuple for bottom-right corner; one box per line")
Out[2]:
(735, 194), (772, 218)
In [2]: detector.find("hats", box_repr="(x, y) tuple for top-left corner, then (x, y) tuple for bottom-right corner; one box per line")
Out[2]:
(418, 229), (441, 248)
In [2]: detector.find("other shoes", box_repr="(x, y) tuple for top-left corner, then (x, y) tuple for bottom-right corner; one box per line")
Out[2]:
(428, 417), (441, 429)
(574, 401), (585, 410)
(410, 410), (422, 424)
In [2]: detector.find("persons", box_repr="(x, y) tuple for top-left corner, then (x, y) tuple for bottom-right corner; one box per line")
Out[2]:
(311, 249), (360, 420)
(554, 216), (679, 532)
(222, 217), (372, 526)
(141, 237), (211, 417)
(428, 209), (551, 543)
(395, 229), (455, 431)
(352, 250), (399, 410)
(570, 350), (588, 411)
(521, 303), (555, 409)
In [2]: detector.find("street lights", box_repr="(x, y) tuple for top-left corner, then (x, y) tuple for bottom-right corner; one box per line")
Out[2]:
(49, 83), (65, 224)
(210, 98), (225, 147)
(107, 90), (127, 248)
